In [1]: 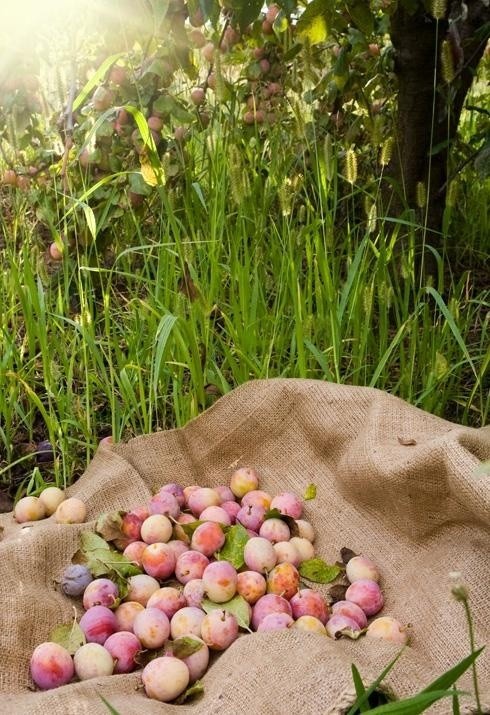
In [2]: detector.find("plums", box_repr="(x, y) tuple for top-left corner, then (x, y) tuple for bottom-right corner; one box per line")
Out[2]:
(2, 7), (381, 261)
(30, 469), (407, 703)
(15, 486), (87, 525)
